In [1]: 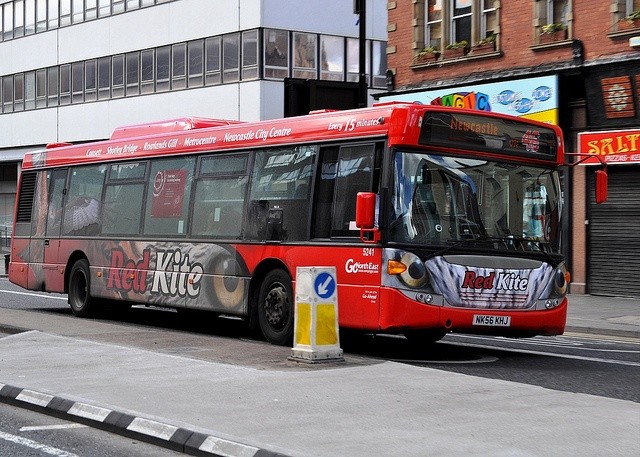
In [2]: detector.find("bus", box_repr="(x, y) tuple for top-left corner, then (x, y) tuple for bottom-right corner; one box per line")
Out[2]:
(8, 101), (608, 345)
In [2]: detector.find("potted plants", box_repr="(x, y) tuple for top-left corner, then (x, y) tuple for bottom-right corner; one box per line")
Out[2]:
(416, 46), (441, 64)
(472, 34), (497, 56)
(617, 9), (640, 31)
(444, 40), (468, 59)
(539, 22), (568, 44)
